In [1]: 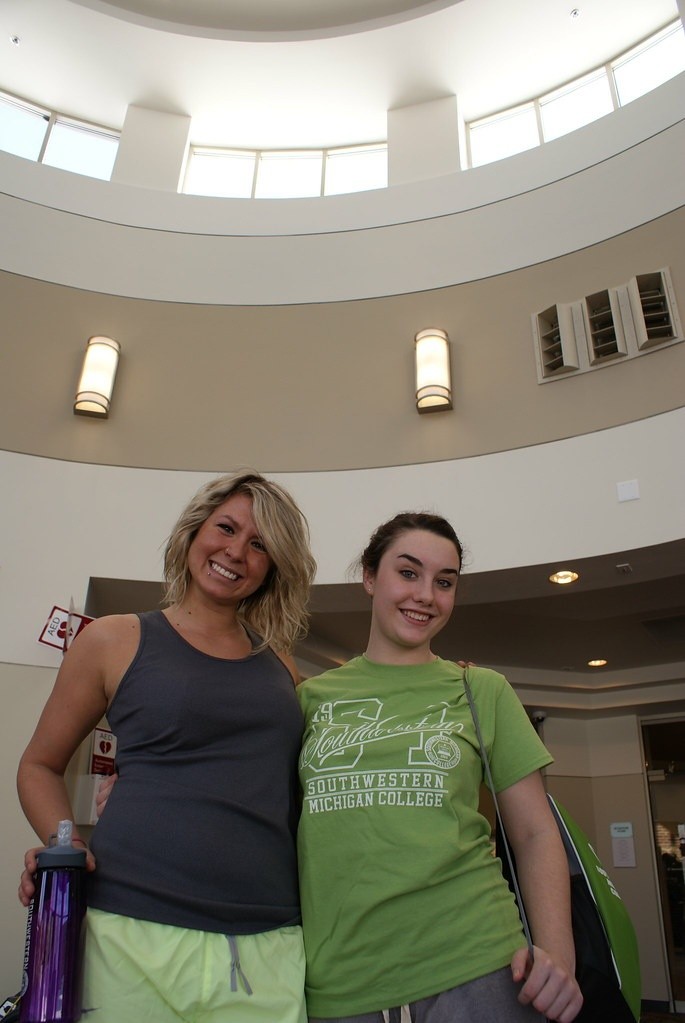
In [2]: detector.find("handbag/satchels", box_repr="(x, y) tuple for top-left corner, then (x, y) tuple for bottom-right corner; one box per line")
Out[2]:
(461, 660), (644, 1023)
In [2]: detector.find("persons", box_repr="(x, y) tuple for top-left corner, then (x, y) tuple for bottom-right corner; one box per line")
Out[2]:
(94, 514), (584, 1023)
(17, 471), (475, 1023)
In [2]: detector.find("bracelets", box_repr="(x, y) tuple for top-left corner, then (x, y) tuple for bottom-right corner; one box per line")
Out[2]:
(72, 835), (88, 847)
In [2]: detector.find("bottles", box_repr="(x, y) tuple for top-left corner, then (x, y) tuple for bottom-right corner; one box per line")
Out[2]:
(18, 820), (87, 1023)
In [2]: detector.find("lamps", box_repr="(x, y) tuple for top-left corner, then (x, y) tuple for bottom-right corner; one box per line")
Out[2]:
(412, 326), (454, 415)
(73, 334), (121, 420)
